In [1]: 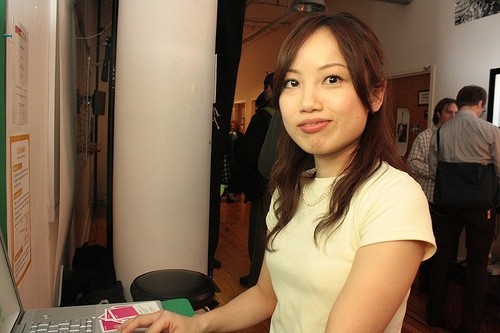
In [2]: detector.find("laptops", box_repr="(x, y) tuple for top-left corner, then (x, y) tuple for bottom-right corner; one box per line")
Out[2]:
(0, 228), (162, 333)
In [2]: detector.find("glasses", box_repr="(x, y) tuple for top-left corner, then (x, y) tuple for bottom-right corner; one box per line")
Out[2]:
(267, 71), (272, 75)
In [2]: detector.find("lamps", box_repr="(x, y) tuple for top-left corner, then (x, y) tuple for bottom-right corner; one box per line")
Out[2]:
(291, 0), (329, 16)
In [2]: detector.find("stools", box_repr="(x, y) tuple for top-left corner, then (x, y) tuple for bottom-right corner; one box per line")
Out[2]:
(130, 269), (221, 313)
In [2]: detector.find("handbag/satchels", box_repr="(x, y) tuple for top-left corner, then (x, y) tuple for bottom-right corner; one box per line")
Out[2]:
(432, 161), (498, 209)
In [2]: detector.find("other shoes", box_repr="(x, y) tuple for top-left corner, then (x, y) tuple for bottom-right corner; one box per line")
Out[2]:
(213, 259), (220, 267)
(240, 277), (257, 286)
(426, 290), (446, 326)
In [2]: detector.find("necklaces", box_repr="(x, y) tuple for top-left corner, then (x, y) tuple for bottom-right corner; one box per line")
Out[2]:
(302, 169), (337, 206)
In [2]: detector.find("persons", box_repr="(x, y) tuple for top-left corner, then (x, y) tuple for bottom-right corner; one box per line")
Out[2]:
(430, 84), (500, 310)
(239, 70), (315, 287)
(220, 120), (244, 203)
(114, 11), (438, 333)
(408, 97), (457, 215)
